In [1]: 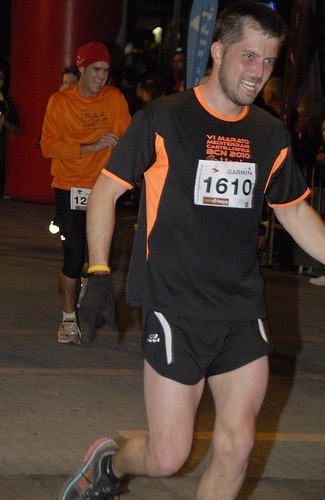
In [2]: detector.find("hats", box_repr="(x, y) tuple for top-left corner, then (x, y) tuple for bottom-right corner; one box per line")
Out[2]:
(76, 42), (110, 67)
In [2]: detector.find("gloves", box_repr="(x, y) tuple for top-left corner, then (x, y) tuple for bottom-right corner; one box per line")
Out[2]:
(79, 272), (118, 342)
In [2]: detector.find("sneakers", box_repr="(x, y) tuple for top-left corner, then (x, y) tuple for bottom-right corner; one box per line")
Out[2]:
(310, 275), (325, 285)
(58, 317), (81, 343)
(60, 437), (123, 500)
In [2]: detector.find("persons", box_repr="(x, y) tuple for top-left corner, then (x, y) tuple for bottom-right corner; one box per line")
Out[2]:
(60, 3), (325, 500)
(0, 31), (325, 344)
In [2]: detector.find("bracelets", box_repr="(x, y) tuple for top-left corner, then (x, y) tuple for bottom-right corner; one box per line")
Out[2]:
(87, 264), (110, 274)
